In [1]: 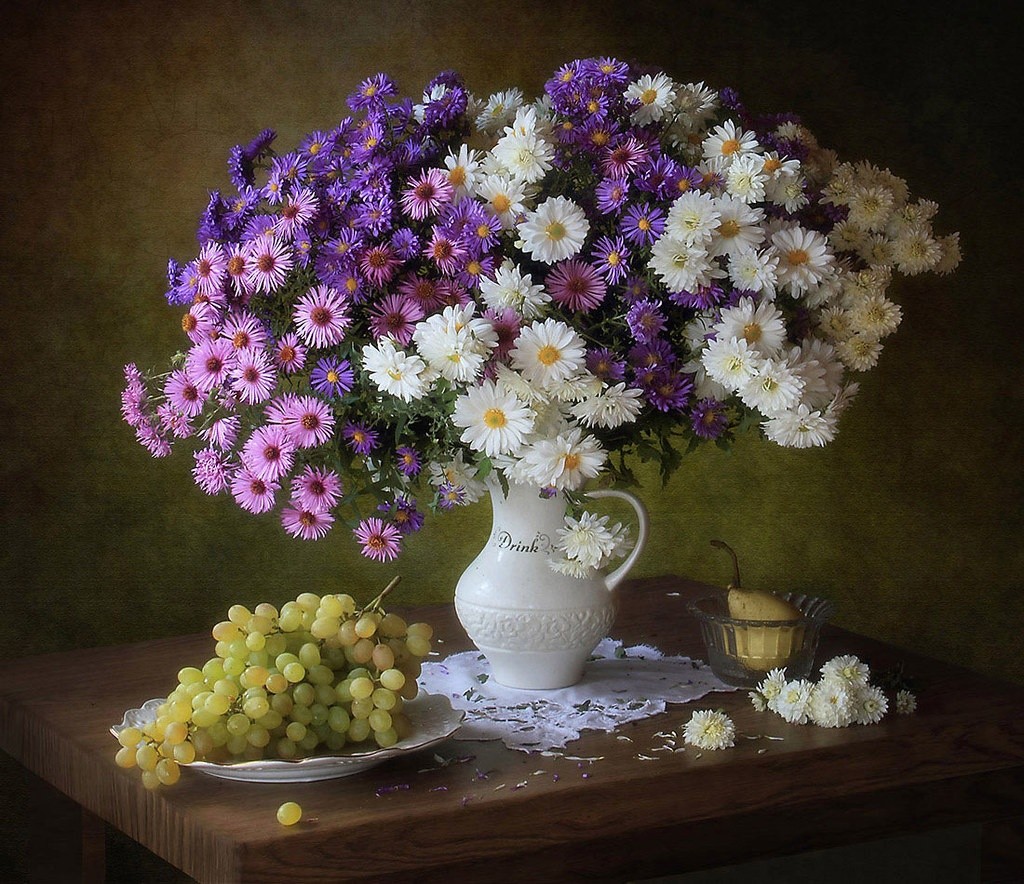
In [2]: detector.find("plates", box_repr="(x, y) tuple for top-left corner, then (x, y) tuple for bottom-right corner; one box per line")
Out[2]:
(110, 687), (466, 784)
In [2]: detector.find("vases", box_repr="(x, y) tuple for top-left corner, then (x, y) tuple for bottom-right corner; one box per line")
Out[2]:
(453, 465), (649, 690)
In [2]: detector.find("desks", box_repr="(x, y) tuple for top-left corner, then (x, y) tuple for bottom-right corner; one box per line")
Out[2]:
(0, 575), (1024, 884)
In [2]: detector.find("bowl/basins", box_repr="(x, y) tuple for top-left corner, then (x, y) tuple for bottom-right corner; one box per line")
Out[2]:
(685, 590), (842, 689)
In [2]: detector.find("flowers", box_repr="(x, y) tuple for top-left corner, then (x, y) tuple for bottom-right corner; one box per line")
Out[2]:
(120, 56), (965, 580)
(684, 655), (919, 750)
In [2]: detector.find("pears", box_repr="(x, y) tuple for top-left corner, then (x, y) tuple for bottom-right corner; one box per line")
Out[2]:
(709, 540), (809, 670)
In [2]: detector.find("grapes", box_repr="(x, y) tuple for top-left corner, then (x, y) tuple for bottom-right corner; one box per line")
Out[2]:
(113, 594), (434, 790)
(275, 801), (302, 826)
